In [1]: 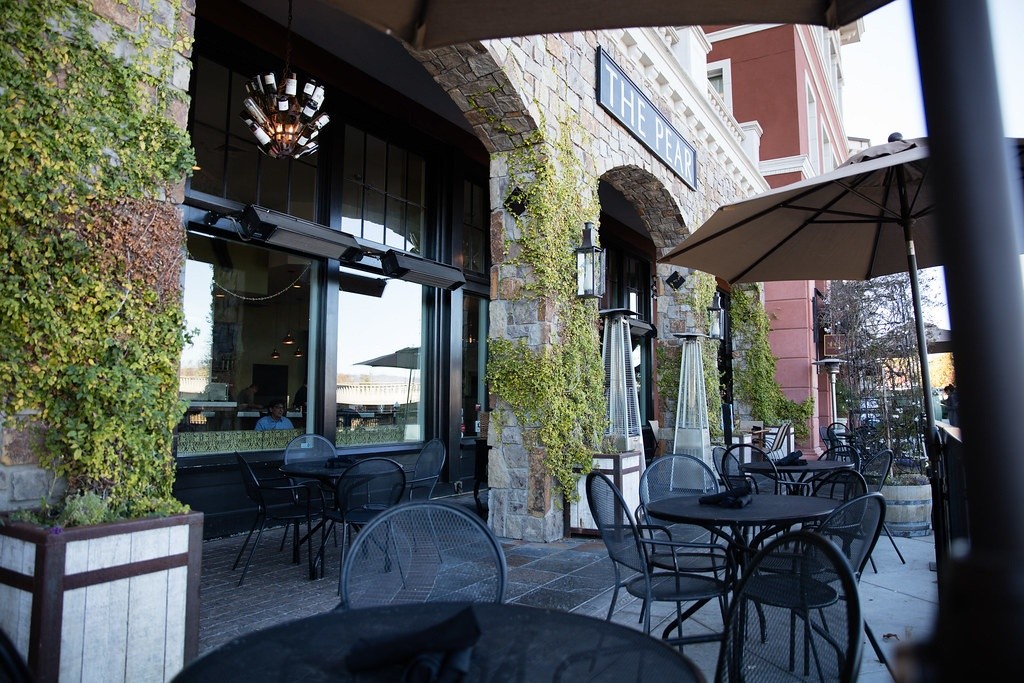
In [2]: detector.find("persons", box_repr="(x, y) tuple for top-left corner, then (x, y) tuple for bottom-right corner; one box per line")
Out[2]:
(293, 377), (307, 412)
(940, 384), (958, 428)
(254, 397), (293, 431)
(236, 384), (263, 408)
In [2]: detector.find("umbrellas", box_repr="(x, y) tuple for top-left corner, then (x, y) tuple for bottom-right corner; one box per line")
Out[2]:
(333, 0), (893, 51)
(354, 347), (421, 429)
(658, 131), (1024, 480)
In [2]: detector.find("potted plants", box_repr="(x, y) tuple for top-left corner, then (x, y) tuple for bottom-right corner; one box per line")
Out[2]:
(657, 267), (753, 479)
(476, 96), (643, 537)
(1, 0), (205, 683)
(728, 284), (814, 460)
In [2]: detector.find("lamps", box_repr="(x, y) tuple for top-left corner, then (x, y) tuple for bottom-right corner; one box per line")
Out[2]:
(576, 222), (606, 300)
(206, 203), (360, 264)
(271, 298), (303, 358)
(359, 246), (466, 293)
(240, 0), (331, 160)
(709, 292), (725, 340)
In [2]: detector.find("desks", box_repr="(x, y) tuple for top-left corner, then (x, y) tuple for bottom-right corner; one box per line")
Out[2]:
(836, 432), (853, 444)
(645, 495), (843, 644)
(180, 402), (303, 430)
(168, 601), (709, 683)
(279, 461), (403, 580)
(736, 462), (856, 550)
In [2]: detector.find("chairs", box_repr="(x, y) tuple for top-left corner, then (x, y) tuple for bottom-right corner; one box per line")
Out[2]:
(230, 420), (908, 683)
